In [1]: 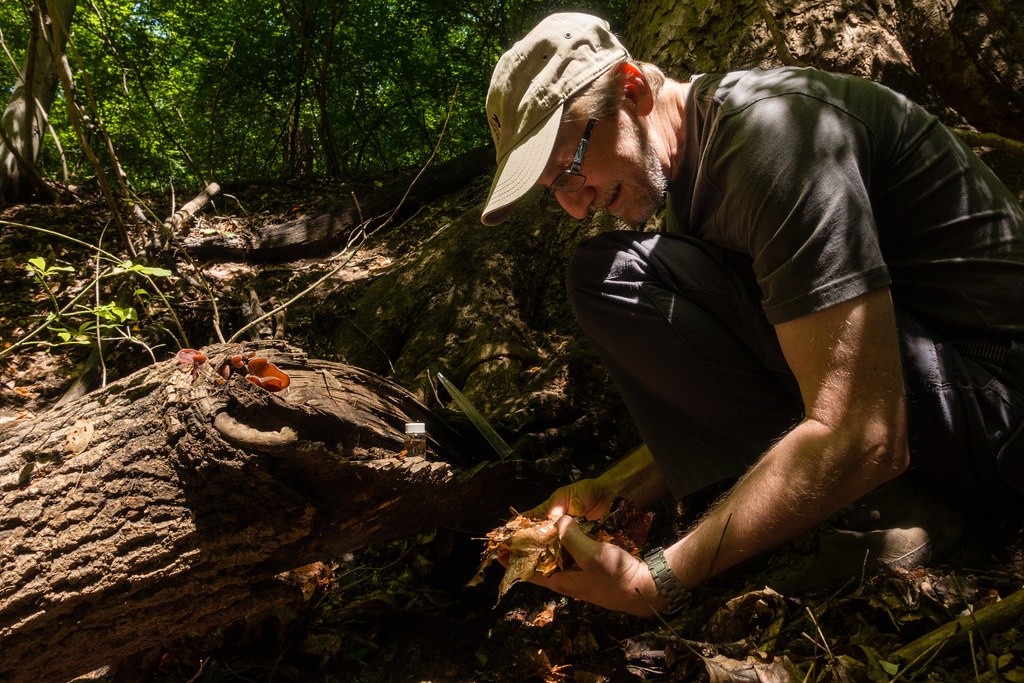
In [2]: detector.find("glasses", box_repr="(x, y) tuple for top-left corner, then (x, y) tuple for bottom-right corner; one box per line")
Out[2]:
(536, 115), (599, 213)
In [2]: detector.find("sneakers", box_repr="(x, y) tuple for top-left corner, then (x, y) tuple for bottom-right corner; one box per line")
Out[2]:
(715, 492), (940, 604)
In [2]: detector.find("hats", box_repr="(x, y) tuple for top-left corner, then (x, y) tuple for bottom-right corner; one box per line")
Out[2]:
(479, 12), (629, 227)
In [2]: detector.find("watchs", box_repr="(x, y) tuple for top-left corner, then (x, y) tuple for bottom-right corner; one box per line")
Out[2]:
(643, 547), (700, 620)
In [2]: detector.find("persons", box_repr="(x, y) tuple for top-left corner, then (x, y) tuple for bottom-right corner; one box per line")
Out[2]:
(467, 13), (1024, 620)
